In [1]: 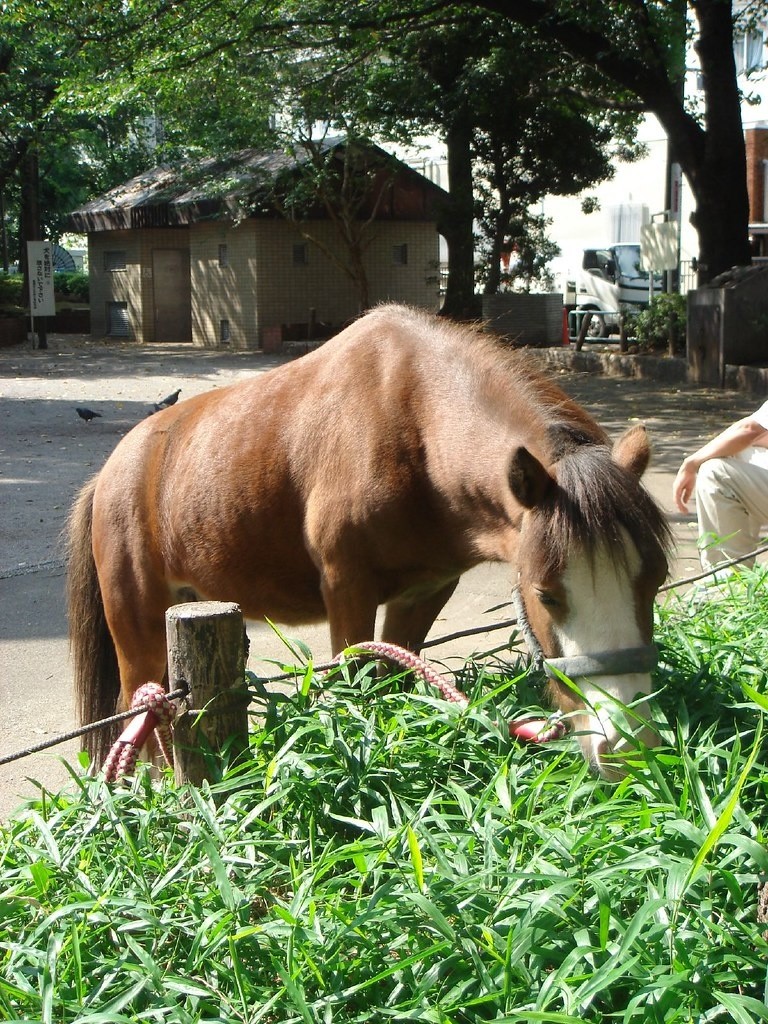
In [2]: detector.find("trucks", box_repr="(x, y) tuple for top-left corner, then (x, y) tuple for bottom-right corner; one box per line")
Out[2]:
(552, 242), (698, 345)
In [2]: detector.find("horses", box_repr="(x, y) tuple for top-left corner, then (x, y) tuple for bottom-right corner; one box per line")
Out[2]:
(57, 298), (683, 790)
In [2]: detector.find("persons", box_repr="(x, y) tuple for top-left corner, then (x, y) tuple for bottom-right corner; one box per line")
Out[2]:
(671, 397), (767, 606)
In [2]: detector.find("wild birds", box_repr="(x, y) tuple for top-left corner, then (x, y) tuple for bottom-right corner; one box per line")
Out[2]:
(75, 406), (103, 422)
(144, 388), (183, 416)
